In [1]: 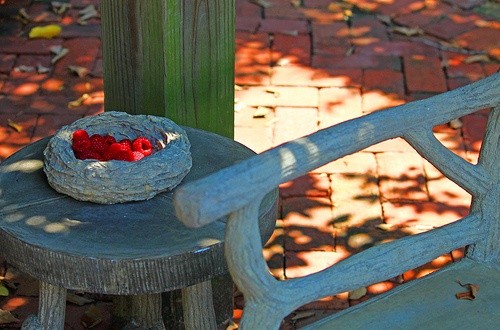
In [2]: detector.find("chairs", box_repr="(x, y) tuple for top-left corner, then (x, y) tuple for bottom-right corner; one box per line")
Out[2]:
(173, 56), (500, 330)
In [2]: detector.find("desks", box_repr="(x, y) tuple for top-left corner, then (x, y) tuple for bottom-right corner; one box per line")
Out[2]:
(0, 124), (283, 330)
